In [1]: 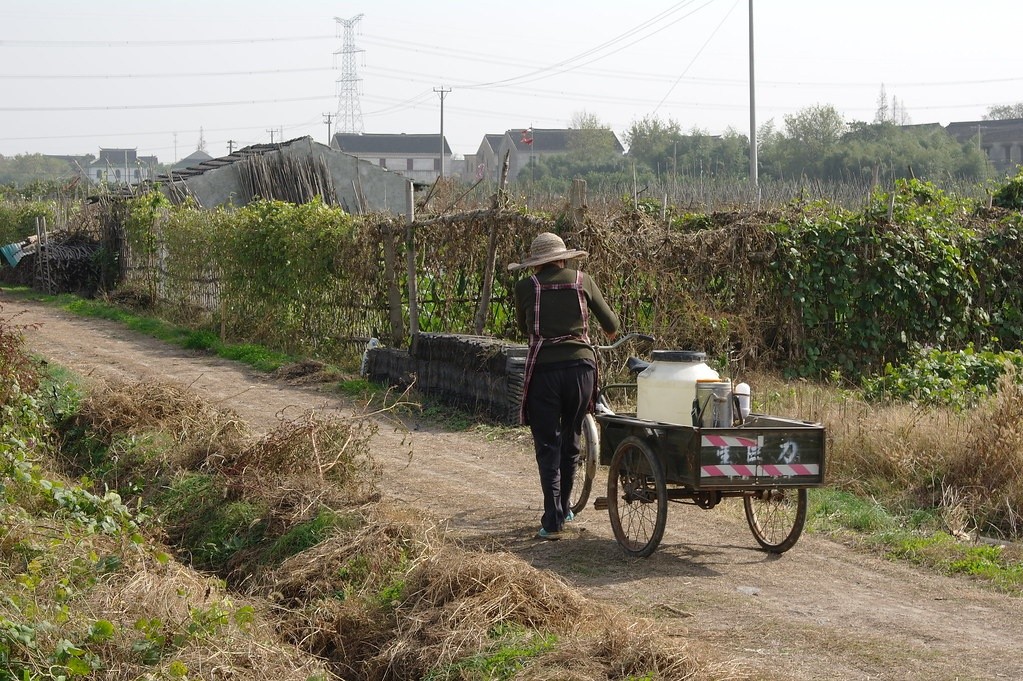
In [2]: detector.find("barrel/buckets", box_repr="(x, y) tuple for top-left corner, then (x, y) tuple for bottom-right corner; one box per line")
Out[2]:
(636, 350), (719, 425)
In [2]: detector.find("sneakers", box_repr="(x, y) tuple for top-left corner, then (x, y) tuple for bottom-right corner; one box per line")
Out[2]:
(563, 509), (574, 522)
(538, 528), (564, 540)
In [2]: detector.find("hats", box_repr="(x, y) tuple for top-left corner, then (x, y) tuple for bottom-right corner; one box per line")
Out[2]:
(506, 231), (590, 273)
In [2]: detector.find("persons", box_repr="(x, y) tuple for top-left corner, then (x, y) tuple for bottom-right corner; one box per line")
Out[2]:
(505, 232), (619, 541)
